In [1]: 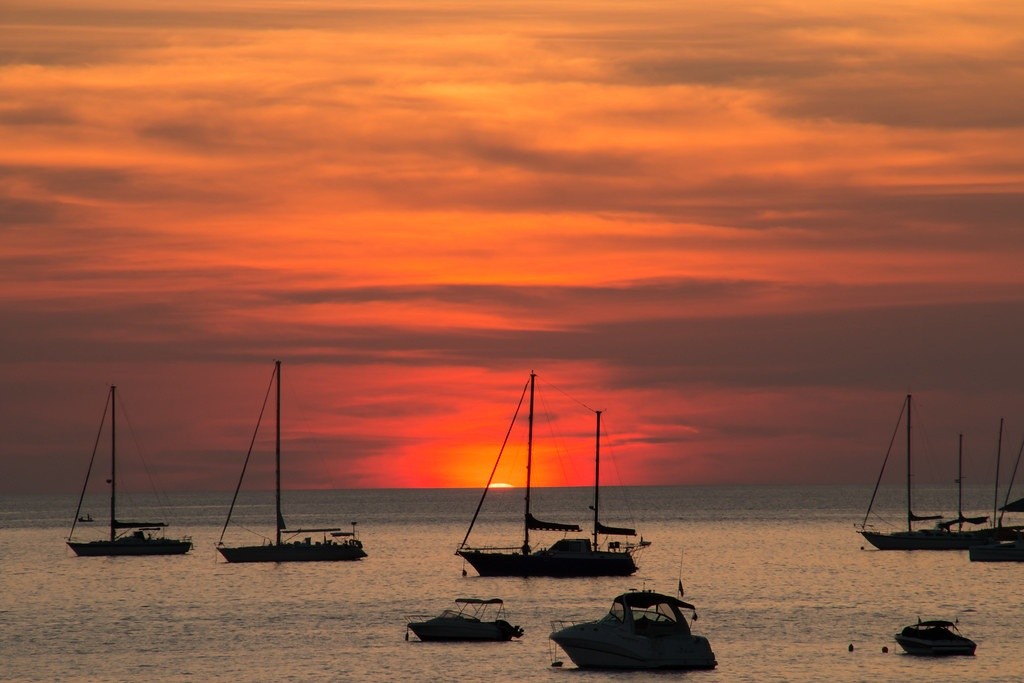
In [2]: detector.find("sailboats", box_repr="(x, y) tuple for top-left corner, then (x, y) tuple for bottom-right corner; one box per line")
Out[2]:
(855, 396), (1024, 563)
(215, 360), (370, 564)
(455, 373), (644, 578)
(65, 384), (194, 557)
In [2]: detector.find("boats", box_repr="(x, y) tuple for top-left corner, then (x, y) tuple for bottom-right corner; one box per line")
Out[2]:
(893, 620), (979, 656)
(550, 591), (719, 672)
(403, 597), (525, 645)
